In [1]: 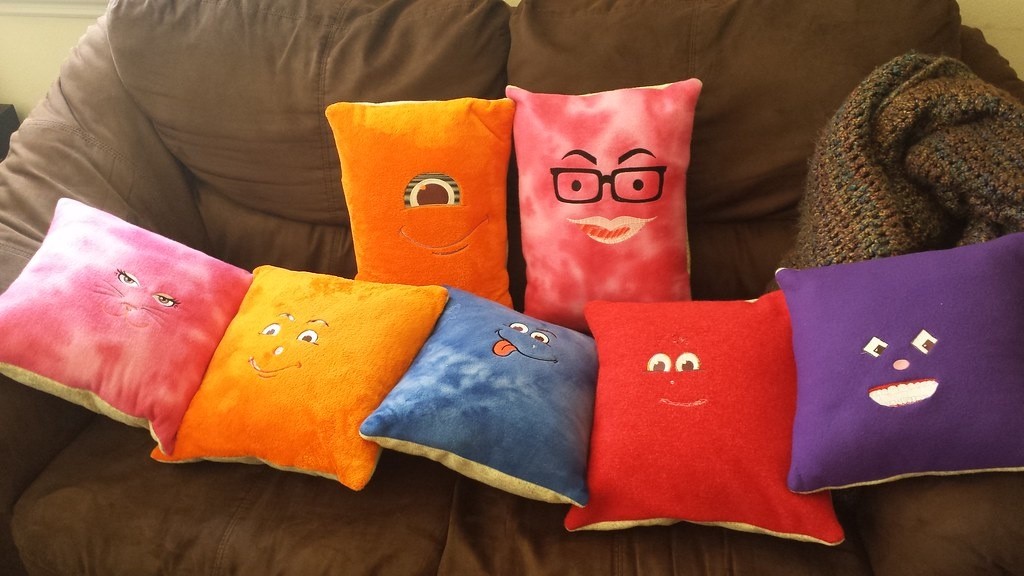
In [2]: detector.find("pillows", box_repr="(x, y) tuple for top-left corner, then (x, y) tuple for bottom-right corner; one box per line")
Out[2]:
(324, 97), (516, 312)
(148, 264), (450, 491)
(358, 286), (598, 507)
(562, 292), (846, 548)
(498, 77), (704, 333)
(773, 231), (1024, 494)
(0, 195), (249, 454)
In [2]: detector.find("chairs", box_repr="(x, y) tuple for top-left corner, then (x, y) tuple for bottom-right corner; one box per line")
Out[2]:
(0, 0), (1024, 576)
